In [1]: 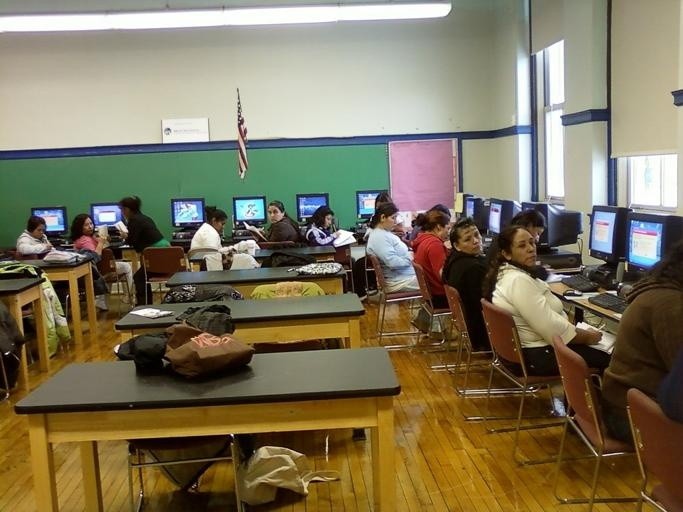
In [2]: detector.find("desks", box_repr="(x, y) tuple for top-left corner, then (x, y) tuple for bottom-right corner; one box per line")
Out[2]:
(0, 234), (401, 511)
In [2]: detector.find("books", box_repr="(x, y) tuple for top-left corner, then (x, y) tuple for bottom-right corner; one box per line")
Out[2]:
(97, 224), (112, 249)
(114, 220), (129, 242)
(330, 229), (357, 248)
(243, 222), (268, 242)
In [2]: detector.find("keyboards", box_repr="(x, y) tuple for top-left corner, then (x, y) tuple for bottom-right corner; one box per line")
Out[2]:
(588, 292), (631, 316)
(562, 274), (602, 293)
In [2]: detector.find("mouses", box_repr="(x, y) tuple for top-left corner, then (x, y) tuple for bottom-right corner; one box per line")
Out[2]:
(563, 289), (582, 296)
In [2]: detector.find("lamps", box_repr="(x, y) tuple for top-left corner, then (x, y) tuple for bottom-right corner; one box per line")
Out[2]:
(0, 2), (452, 33)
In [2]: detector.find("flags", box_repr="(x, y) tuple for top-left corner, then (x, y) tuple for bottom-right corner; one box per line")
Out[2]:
(237, 97), (249, 180)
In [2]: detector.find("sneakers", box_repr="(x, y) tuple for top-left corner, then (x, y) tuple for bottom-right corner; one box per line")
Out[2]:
(120, 294), (137, 304)
(410, 318), (457, 340)
(94, 295), (107, 310)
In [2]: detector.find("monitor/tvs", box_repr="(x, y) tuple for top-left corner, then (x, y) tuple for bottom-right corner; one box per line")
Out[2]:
(520, 202), (582, 254)
(30, 206), (68, 238)
(356, 190), (389, 224)
(232, 194), (267, 229)
(587, 205), (635, 271)
(487, 198), (521, 243)
(171, 199), (206, 232)
(296, 193), (329, 225)
(627, 212), (683, 280)
(91, 202), (127, 234)
(463, 197), (493, 235)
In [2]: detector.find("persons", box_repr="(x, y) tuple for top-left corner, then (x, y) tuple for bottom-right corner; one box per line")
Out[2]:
(16, 216), (56, 255)
(260, 200), (301, 268)
(600, 231), (682, 443)
(301, 205), (341, 246)
(482, 225), (611, 436)
(118, 195), (171, 307)
(70, 214), (138, 311)
(363, 193), (550, 350)
(189, 206), (234, 260)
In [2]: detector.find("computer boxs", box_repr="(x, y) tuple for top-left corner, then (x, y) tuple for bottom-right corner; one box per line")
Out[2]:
(538, 251), (582, 268)
(231, 229), (266, 237)
(172, 232), (198, 241)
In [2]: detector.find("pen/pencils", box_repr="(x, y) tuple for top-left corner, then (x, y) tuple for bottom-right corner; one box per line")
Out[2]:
(287, 268), (296, 272)
(596, 323), (606, 331)
(332, 224), (337, 231)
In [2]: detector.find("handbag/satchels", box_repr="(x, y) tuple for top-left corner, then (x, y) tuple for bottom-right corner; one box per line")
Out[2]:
(43, 251), (86, 263)
(163, 320), (254, 378)
(295, 263), (341, 275)
(235, 446), (311, 507)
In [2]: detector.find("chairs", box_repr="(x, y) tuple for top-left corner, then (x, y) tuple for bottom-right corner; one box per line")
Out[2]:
(364, 245), (683, 512)
